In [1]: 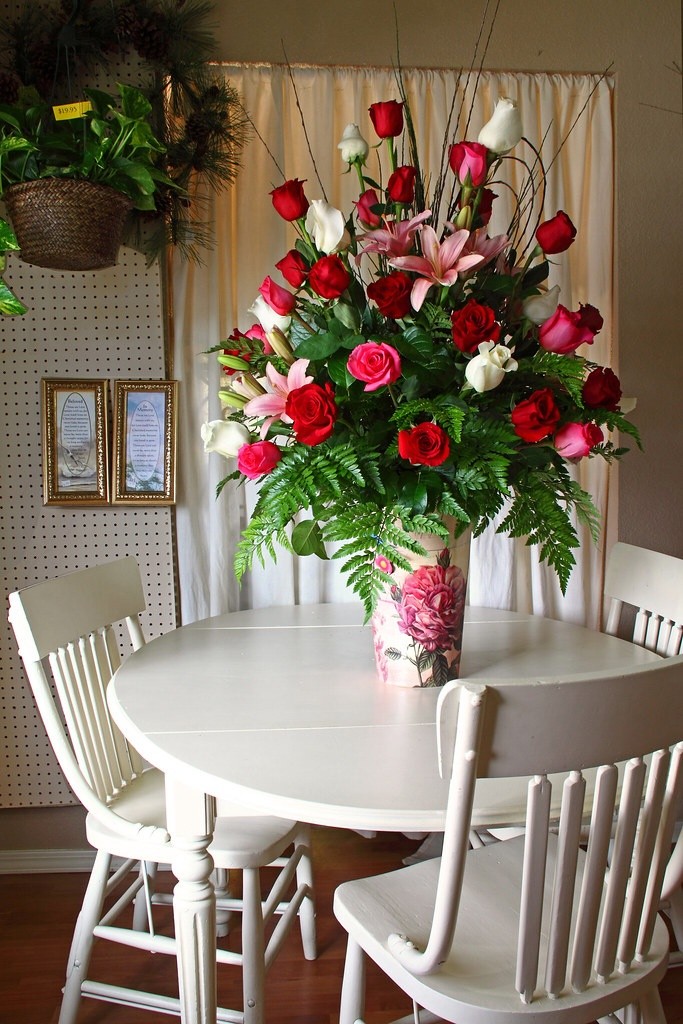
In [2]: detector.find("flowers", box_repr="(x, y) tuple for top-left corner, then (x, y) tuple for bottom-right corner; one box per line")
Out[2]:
(215, 0), (649, 625)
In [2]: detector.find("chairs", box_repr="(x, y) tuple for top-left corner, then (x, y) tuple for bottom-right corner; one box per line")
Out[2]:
(1, 541), (683, 1024)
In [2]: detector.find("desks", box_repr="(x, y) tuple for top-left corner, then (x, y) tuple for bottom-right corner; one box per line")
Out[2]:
(105, 603), (683, 1024)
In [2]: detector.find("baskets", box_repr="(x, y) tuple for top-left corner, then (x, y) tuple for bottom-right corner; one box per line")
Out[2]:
(3, 180), (132, 271)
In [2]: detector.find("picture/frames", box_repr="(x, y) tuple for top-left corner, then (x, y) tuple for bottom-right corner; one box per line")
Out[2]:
(40, 377), (113, 507)
(112, 379), (180, 507)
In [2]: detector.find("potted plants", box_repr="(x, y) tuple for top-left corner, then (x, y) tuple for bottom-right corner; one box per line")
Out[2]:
(0, 80), (191, 316)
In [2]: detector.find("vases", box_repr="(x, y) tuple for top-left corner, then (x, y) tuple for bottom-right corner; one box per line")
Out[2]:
(372, 508), (473, 688)
